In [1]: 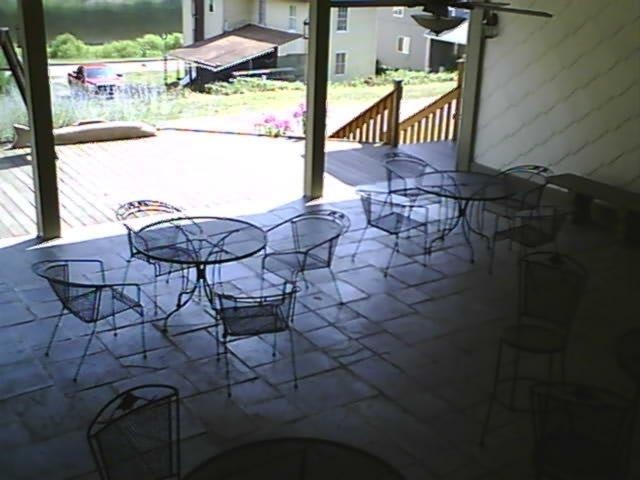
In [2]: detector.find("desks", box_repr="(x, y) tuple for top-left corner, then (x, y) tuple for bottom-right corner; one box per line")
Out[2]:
(131, 217), (267, 362)
(415, 171), (523, 264)
(182, 437), (404, 480)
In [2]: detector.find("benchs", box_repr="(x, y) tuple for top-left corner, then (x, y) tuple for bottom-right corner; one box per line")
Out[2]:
(547, 173), (640, 243)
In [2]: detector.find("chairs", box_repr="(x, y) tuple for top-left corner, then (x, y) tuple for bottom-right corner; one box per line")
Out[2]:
(479, 251), (579, 448)
(86, 384), (181, 480)
(525, 380), (635, 479)
(488, 204), (567, 273)
(31, 259), (146, 382)
(260, 209), (351, 323)
(211, 283), (302, 398)
(117, 200), (203, 316)
(381, 152), (445, 232)
(351, 189), (429, 278)
(481, 163), (554, 250)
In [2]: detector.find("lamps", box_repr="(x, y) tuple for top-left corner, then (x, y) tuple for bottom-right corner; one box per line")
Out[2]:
(482, 13), (498, 38)
(410, 3), (466, 35)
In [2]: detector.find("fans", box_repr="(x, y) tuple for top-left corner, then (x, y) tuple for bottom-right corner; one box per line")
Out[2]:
(332, 0), (555, 35)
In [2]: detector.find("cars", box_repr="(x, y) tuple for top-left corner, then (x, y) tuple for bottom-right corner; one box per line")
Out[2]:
(67, 62), (130, 100)
